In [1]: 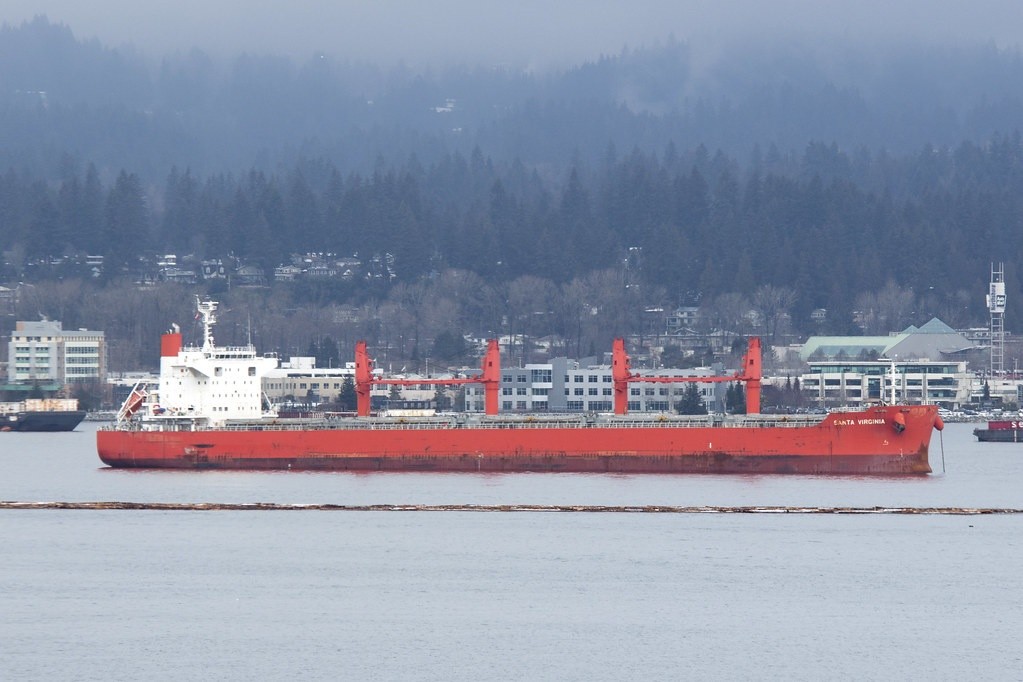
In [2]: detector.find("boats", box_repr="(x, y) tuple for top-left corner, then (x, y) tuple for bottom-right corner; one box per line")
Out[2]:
(0, 398), (87, 432)
(123, 390), (146, 418)
(973, 420), (1023, 443)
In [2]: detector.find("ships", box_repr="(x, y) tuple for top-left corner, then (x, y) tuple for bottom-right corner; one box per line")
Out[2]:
(97, 294), (943, 475)
